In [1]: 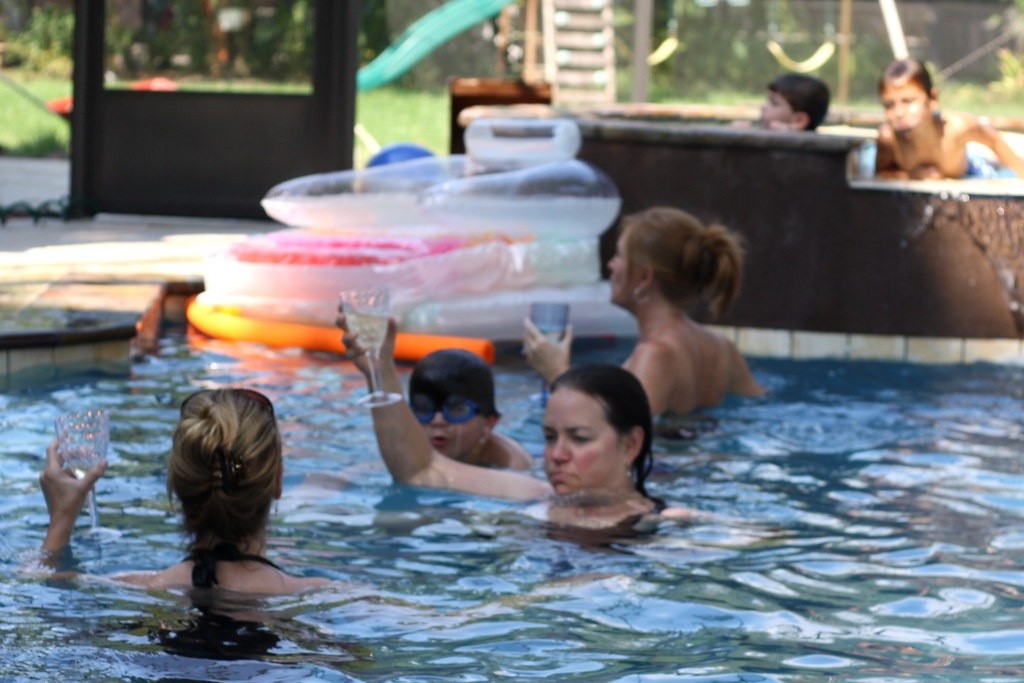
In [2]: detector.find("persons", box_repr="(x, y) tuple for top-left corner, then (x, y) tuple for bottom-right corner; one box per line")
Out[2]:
(730, 72), (830, 131)
(408, 348), (533, 470)
(39, 389), (332, 594)
(523, 208), (766, 422)
(876, 58), (1024, 180)
(335, 301), (698, 518)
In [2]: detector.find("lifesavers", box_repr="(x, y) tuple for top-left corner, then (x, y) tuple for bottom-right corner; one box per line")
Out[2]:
(184, 118), (640, 363)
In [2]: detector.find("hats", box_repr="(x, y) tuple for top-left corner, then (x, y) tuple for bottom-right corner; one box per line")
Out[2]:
(408, 348), (495, 416)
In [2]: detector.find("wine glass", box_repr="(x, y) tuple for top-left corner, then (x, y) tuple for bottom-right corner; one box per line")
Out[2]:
(57, 409), (122, 544)
(340, 286), (403, 409)
(530, 303), (567, 402)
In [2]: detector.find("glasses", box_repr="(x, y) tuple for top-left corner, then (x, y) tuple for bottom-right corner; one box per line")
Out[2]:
(180, 388), (274, 415)
(411, 392), (478, 423)
